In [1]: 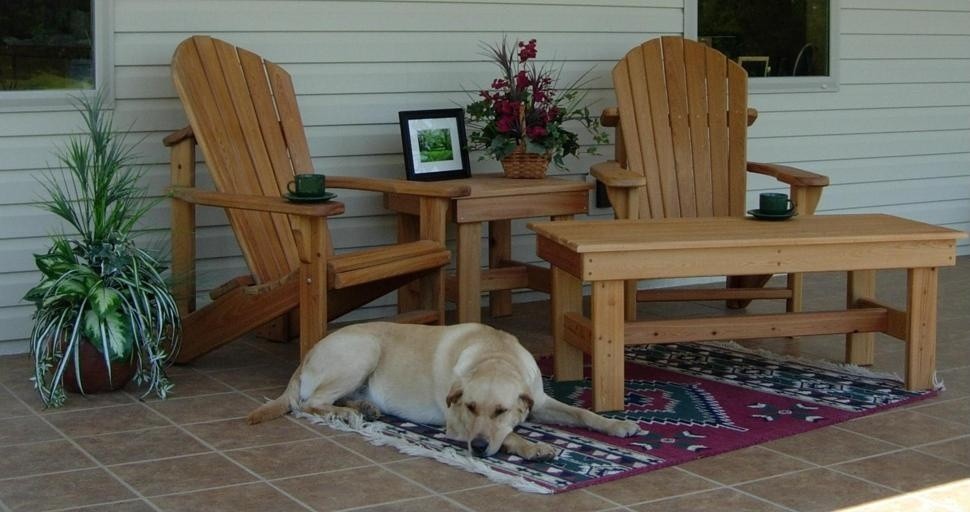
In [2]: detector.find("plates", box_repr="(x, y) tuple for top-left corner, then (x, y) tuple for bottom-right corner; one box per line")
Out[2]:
(282, 191), (338, 203)
(747, 208), (799, 220)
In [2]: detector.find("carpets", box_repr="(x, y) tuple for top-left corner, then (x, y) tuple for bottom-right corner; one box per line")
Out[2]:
(292, 334), (944, 495)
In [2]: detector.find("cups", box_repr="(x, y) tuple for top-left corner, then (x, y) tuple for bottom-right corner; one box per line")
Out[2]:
(287, 172), (327, 196)
(758, 192), (795, 212)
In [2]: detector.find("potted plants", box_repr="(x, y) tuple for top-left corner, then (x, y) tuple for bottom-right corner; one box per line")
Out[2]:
(19, 83), (179, 407)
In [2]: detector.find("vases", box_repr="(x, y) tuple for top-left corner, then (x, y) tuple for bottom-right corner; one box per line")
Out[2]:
(499, 151), (549, 181)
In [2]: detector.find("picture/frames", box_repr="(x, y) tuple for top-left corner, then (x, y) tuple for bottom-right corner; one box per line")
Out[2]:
(395, 105), (471, 182)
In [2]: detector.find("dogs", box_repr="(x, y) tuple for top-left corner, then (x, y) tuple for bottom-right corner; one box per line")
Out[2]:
(246, 320), (641, 463)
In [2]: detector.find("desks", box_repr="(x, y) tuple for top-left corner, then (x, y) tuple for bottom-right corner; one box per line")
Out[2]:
(525, 210), (968, 414)
(382, 175), (596, 326)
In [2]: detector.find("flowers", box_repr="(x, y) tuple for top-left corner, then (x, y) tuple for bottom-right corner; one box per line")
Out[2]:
(459, 32), (608, 164)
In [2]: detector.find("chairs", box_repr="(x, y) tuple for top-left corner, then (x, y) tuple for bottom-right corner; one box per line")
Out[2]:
(157, 31), (476, 363)
(589, 32), (831, 339)
